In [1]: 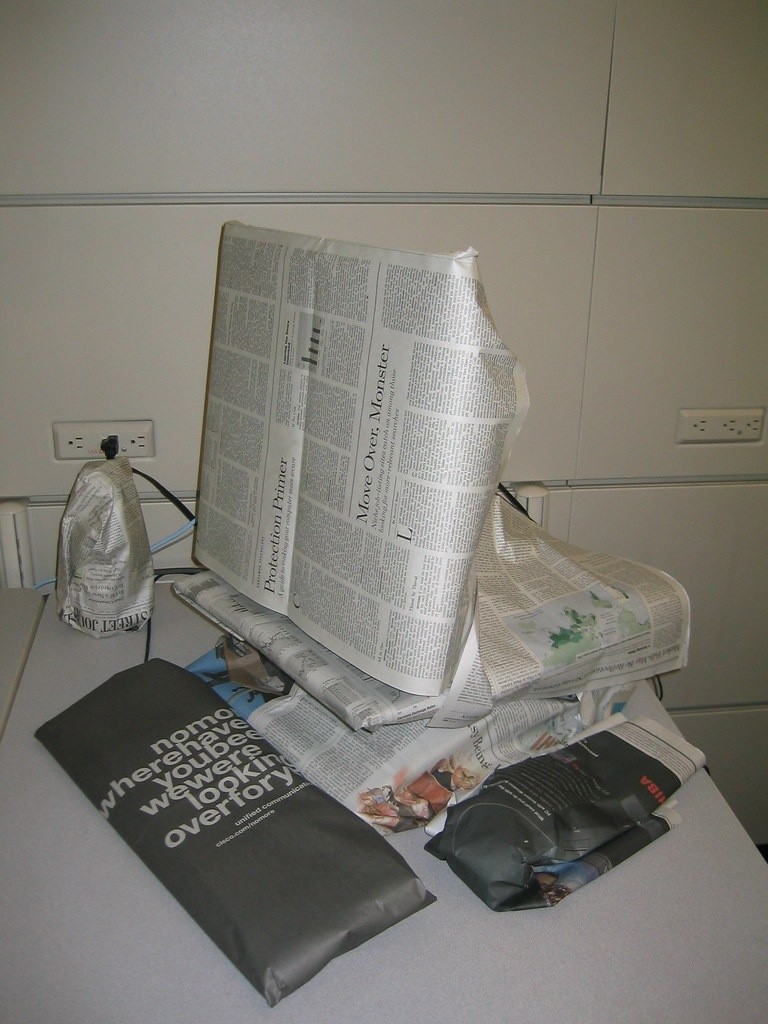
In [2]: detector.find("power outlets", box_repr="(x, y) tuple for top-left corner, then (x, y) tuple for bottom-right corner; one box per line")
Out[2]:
(673, 407), (766, 445)
(51, 420), (156, 460)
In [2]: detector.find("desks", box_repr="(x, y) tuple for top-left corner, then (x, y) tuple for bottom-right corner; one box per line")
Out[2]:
(0, 584), (768, 1024)
